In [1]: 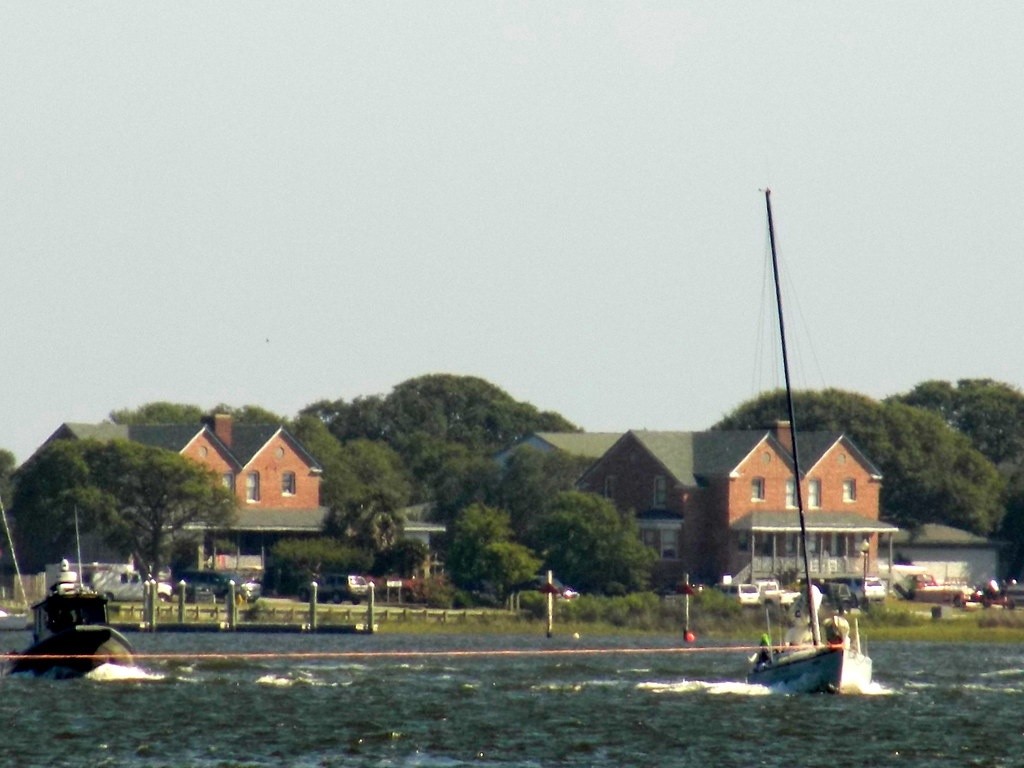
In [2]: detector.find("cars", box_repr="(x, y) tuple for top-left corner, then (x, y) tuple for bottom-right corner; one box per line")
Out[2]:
(902, 573), (937, 590)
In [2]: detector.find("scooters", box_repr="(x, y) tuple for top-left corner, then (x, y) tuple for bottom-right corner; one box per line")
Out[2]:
(980, 580), (1017, 610)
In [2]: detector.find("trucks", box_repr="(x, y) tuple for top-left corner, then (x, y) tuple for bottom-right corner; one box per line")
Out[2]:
(749, 581), (802, 610)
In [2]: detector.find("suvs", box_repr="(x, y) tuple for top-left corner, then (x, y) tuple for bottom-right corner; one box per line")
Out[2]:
(170, 569), (262, 604)
(91, 569), (173, 603)
(826, 576), (886, 603)
(297, 574), (369, 605)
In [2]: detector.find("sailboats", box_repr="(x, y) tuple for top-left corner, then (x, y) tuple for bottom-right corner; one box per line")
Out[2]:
(746, 189), (877, 695)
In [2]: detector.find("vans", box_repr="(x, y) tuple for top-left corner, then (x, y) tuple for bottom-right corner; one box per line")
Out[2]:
(712, 583), (761, 609)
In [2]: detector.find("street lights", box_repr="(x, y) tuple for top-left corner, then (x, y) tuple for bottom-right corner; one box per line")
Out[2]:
(859, 538), (870, 606)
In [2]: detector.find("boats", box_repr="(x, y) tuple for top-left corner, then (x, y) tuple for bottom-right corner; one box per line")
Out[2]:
(0, 500), (136, 670)
